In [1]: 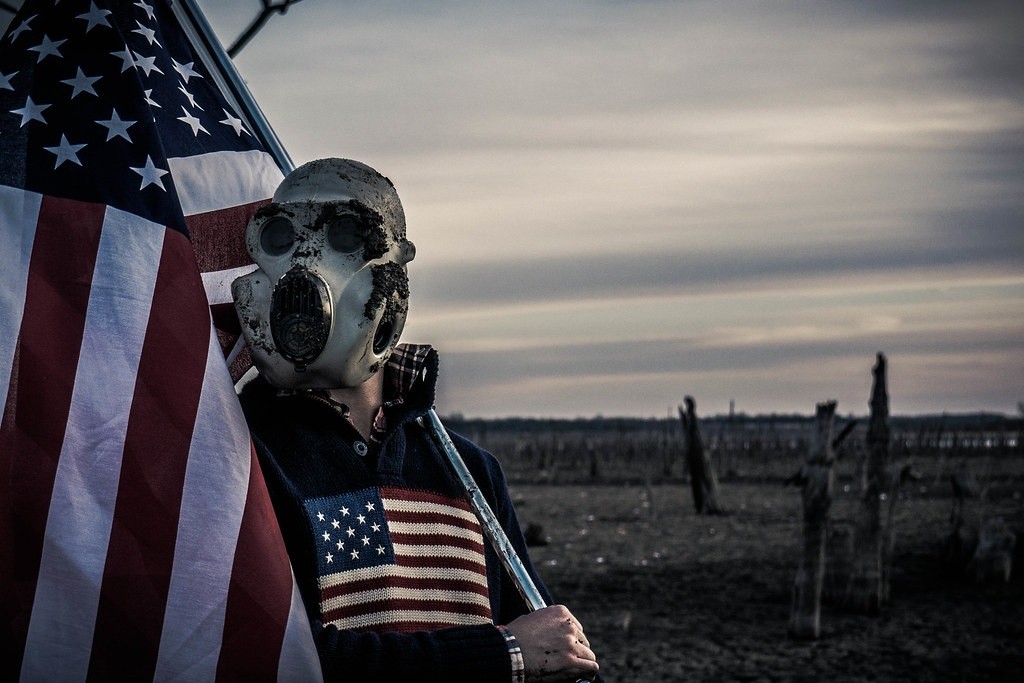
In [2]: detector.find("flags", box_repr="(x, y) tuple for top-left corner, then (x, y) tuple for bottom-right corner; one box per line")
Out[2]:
(0, 0), (323, 683)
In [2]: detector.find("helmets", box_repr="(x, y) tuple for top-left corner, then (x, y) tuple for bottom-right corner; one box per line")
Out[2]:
(230, 158), (416, 388)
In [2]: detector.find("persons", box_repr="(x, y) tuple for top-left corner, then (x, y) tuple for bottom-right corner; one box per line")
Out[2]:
(230, 160), (599, 683)
